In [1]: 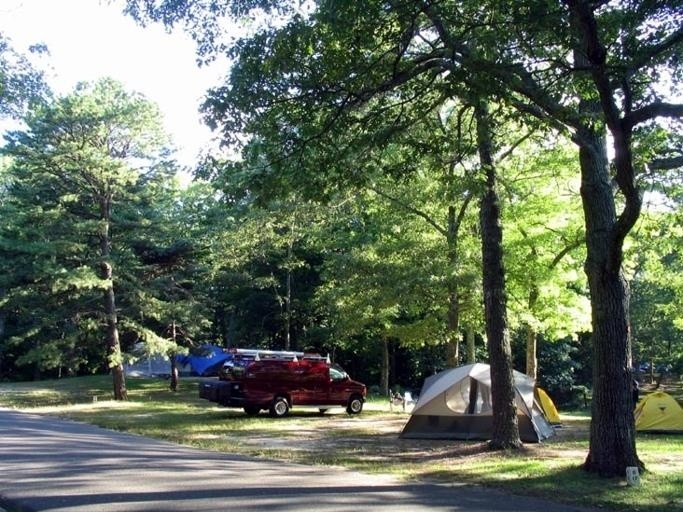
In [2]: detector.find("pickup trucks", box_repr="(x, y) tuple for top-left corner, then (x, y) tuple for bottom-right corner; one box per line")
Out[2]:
(234, 355), (368, 415)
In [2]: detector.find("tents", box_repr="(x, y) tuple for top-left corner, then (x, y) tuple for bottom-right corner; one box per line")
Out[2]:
(399, 362), (554, 444)
(533, 386), (562, 427)
(632, 392), (683, 434)
